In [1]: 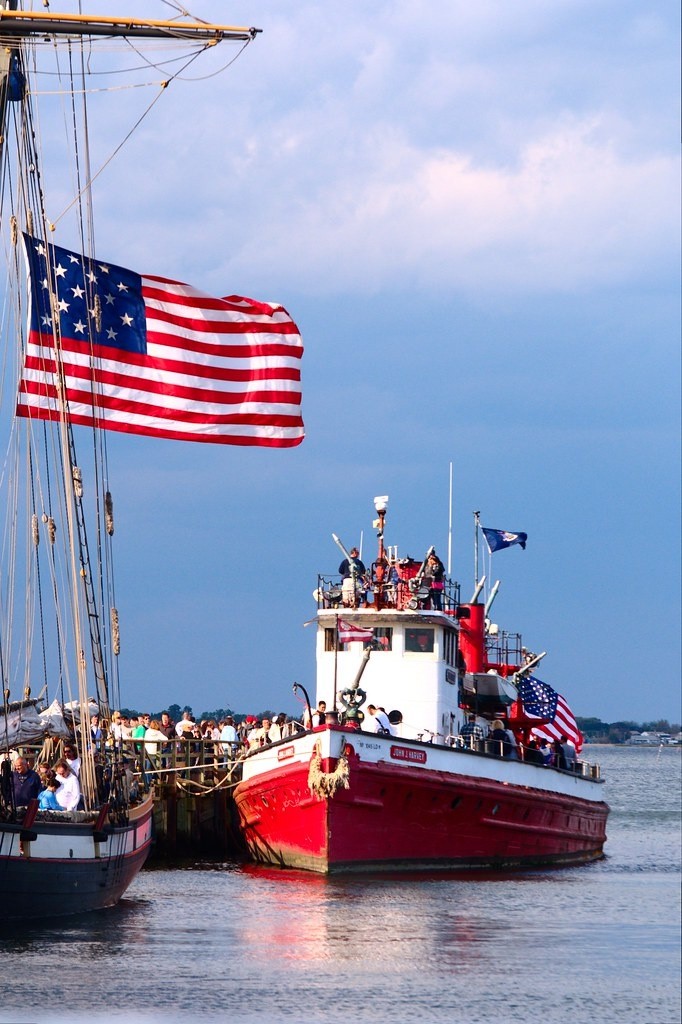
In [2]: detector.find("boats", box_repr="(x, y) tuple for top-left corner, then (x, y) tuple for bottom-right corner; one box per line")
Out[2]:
(225, 459), (614, 885)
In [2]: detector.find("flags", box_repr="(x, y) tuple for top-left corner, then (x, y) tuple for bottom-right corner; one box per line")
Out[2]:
(338, 618), (373, 642)
(16, 231), (306, 448)
(515, 675), (583, 753)
(483, 528), (528, 553)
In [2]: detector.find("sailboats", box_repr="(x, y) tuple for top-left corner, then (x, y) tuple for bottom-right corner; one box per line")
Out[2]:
(2, 0), (266, 924)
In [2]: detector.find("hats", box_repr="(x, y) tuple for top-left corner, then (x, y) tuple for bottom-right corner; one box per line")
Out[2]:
(262, 717), (269, 720)
(246, 716), (252, 722)
(252, 717), (259, 722)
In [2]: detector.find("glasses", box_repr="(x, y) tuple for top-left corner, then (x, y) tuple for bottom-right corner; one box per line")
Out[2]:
(64, 750), (71, 753)
(118, 717), (121, 719)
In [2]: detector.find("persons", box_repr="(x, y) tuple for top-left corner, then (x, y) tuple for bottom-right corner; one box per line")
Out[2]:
(312, 701), (325, 727)
(460, 714), (517, 757)
(422, 550), (445, 611)
(0, 745), (137, 811)
(526, 736), (577, 769)
(368, 704), (392, 734)
(338, 550), (366, 608)
(66, 711), (301, 784)
